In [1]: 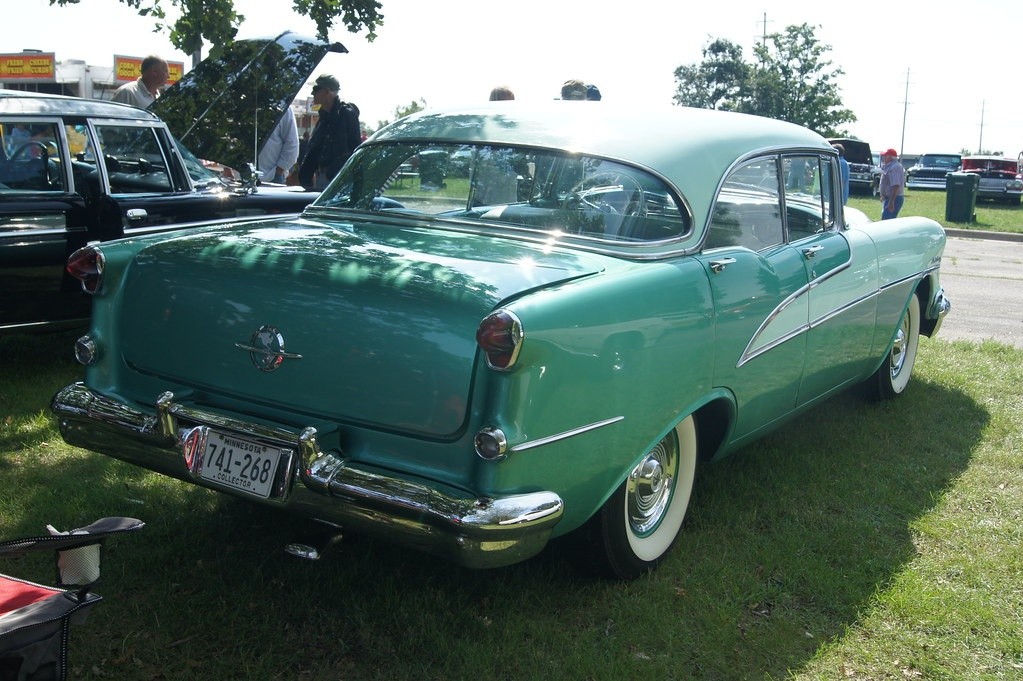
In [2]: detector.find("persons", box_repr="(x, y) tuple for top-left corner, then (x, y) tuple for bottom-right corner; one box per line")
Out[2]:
(300, 74), (363, 198)
(532, 80), (602, 197)
(469, 86), (529, 207)
(879, 149), (904, 221)
(222, 105), (299, 184)
(298, 131), (310, 164)
(102, 55), (169, 142)
(8, 124), (86, 159)
(825, 144), (849, 206)
(360, 131), (368, 142)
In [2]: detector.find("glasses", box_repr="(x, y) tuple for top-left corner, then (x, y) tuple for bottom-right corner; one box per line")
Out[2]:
(314, 86), (324, 90)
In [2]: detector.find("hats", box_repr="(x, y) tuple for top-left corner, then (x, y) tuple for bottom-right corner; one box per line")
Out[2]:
(307, 74), (339, 92)
(880, 149), (897, 157)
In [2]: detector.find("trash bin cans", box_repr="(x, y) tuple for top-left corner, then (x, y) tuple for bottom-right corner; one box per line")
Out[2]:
(945, 172), (980, 224)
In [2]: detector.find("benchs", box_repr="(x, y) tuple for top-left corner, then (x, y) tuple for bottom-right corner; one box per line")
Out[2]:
(480, 207), (758, 249)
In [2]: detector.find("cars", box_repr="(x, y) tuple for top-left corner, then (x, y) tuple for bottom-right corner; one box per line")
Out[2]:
(730, 157), (813, 186)
(451, 144), (474, 168)
(0, 30), (406, 357)
(906, 153), (961, 190)
(826, 137), (877, 192)
(957, 155), (1023, 204)
(54, 98), (949, 580)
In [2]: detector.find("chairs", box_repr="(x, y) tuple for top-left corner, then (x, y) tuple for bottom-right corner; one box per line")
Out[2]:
(0, 515), (147, 681)
